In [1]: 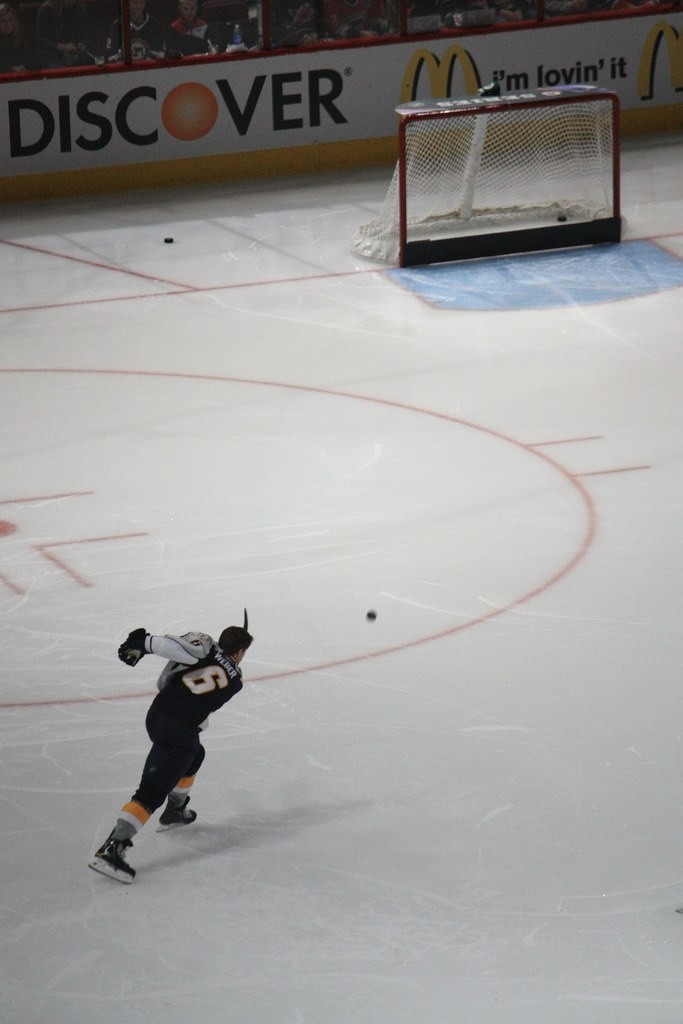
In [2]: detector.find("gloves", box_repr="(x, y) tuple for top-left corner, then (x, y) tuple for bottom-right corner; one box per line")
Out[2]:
(117, 628), (149, 666)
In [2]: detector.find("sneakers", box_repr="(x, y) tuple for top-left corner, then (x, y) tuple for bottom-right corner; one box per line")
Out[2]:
(155, 797), (198, 832)
(87, 832), (136, 884)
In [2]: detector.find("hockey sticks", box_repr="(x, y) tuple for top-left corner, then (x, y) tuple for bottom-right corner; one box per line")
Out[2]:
(243, 608), (248, 638)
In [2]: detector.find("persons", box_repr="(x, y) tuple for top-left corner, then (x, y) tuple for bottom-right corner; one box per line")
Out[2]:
(89, 627), (254, 884)
(1, 0), (548, 74)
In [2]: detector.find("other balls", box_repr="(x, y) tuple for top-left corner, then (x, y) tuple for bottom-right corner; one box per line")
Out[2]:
(164, 237), (174, 243)
(556, 215), (566, 222)
(366, 611), (377, 621)
